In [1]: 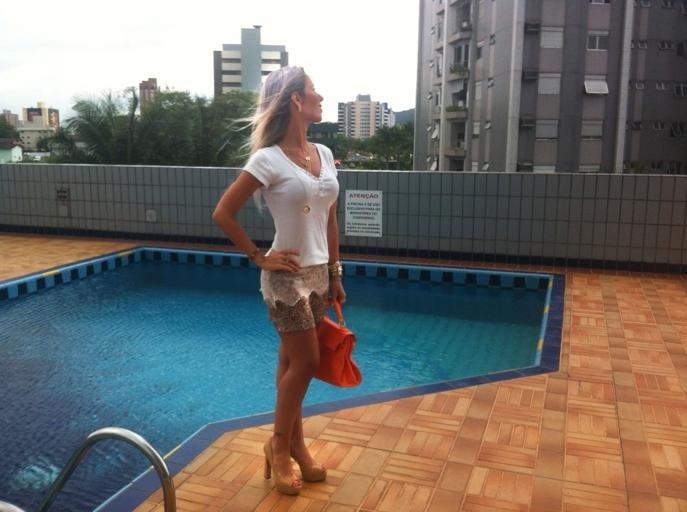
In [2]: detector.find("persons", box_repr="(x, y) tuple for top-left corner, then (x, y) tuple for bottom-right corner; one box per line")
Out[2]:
(210, 65), (349, 497)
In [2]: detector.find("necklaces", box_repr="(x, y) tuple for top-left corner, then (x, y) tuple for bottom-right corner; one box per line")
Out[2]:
(277, 139), (312, 163)
(278, 142), (311, 169)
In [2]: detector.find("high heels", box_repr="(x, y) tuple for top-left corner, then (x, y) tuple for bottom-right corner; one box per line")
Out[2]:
(289, 451), (326, 482)
(263, 438), (303, 495)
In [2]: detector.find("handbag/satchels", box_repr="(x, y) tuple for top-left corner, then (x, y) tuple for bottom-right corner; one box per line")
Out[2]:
(312, 318), (362, 388)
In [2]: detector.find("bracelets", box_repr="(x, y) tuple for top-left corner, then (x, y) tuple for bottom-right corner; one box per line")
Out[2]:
(330, 265), (343, 272)
(247, 246), (261, 262)
(330, 271), (344, 277)
(328, 259), (341, 270)
